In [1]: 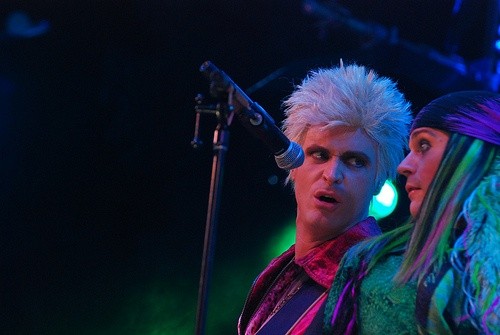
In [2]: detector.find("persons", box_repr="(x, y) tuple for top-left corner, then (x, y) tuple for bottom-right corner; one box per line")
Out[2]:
(235, 59), (415, 335)
(324, 87), (500, 335)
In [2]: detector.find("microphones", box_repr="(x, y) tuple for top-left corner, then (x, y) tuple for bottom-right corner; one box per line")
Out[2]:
(199, 60), (305, 171)
